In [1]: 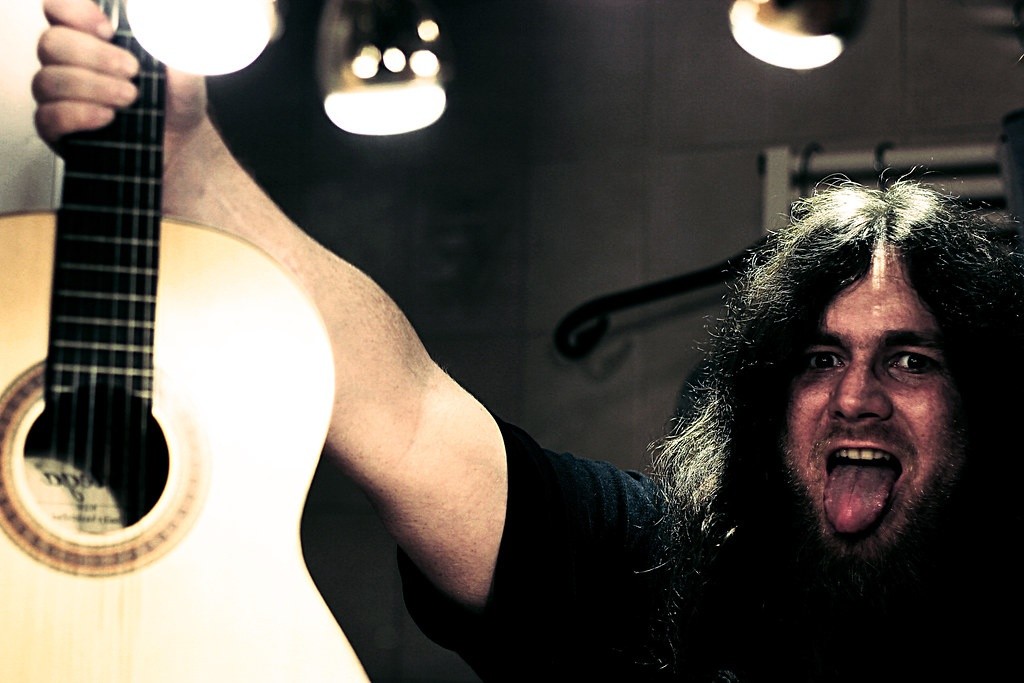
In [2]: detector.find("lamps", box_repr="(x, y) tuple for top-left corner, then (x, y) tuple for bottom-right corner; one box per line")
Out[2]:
(727, 0), (873, 72)
(315, 0), (452, 137)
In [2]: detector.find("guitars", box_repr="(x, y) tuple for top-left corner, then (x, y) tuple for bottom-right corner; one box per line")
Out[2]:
(0, 0), (372, 682)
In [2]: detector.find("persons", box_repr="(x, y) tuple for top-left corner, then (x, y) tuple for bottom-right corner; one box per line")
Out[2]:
(33, 0), (1024, 683)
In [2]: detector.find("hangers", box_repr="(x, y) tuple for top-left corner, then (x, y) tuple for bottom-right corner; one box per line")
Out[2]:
(551, 138), (901, 362)
